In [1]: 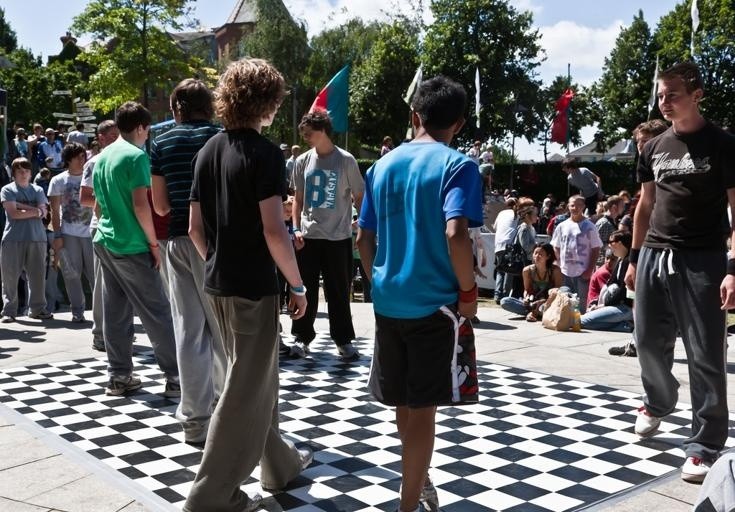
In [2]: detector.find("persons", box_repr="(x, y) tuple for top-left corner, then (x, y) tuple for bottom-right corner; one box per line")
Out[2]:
(92, 100), (181, 398)
(9, 127), (29, 160)
(549, 194), (604, 315)
(47, 141), (94, 323)
(632, 119), (669, 156)
(280, 142), (289, 158)
(380, 135), (393, 156)
(617, 214), (633, 231)
(33, 167), (51, 196)
(504, 189), (511, 197)
(150, 78), (222, 446)
(561, 157), (607, 217)
(595, 201), (608, 216)
(350, 214), (372, 302)
(619, 190), (631, 204)
(546, 204), (571, 237)
(65, 124), (89, 150)
(479, 144), (495, 190)
(286, 145), (301, 183)
(7, 121), (28, 144)
(87, 141), (101, 161)
(356, 75), (485, 512)
(42, 212), (56, 313)
(79, 119), (120, 352)
(21, 141), (23, 144)
(182, 56), (314, 512)
(278, 187), (295, 314)
(0, 114), (9, 167)
(512, 197), (538, 299)
(537, 188), (570, 235)
(351, 194), (358, 218)
(623, 61), (735, 484)
(289, 109), (366, 361)
(37, 127), (65, 177)
(579, 230), (634, 332)
(0, 157), (53, 323)
(467, 140), (482, 161)
(490, 197), (520, 302)
(500, 241), (572, 322)
(595, 195), (624, 271)
(27, 122), (45, 155)
(586, 248), (618, 313)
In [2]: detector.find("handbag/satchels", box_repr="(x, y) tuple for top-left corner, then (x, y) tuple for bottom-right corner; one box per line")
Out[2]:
(540, 288), (578, 331)
(494, 243), (527, 275)
(598, 280), (627, 307)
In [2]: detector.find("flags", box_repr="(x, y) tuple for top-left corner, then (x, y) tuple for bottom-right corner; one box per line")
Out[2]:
(307, 64), (350, 134)
(551, 88), (576, 144)
(401, 62), (424, 112)
(475, 67), (482, 129)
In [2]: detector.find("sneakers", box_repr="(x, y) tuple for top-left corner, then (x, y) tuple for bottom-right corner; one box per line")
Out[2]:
(165, 381), (181, 398)
(525, 311), (538, 321)
(335, 344), (360, 362)
(36, 311), (53, 319)
(290, 342), (310, 359)
(92, 335), (106, 351)
(279, 334), (291, 354)
(634, 404), (663, 438)
(261, 445), (315, 490)
(681, 453), (722, 482)
(397, 501), (429, 512)
(420, 474), (439, 511)
(1, 316), (14, 322)
(245, 490), (262, 512)
(185, 439), (206, 448)
(609, 343), (636, 357)
(105, 375), (143, 396)
(72, 313), (85, 322)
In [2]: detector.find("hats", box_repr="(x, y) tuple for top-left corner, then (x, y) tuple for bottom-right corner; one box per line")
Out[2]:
(505, 197), (518, 207)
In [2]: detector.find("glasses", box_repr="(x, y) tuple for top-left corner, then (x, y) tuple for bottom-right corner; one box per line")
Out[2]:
(299, 129), (314, 137)
(607, 239), (615, 244)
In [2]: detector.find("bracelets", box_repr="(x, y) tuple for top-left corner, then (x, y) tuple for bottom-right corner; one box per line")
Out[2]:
(458, 282), (478, 303)
(39, 207), (44, 217)
(149, 243), (159, 249)
(54, 232), (62, 238)
(290, 285), (307, 296)
(726, 258), (735, 276)
(292, 228), (300, 233)
(628, 248), (641, 263)
(292, 287), (304, 292)
